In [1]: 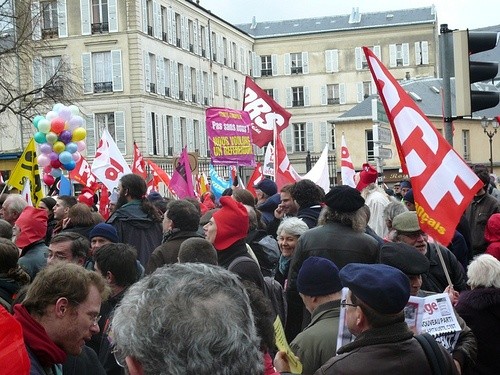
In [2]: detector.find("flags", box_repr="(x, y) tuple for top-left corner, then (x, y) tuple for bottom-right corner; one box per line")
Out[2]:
(7, 138), (43, 208)
(47, 127), (356, 220)
(242, 77), (292, 148)
(362, 47), (484, 246)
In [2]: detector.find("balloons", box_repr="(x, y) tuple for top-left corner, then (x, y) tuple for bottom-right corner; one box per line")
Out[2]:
(32, 103), (86, 185)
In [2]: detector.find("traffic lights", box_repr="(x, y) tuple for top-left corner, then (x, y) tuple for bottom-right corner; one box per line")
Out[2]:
(453, 28), (500, 118)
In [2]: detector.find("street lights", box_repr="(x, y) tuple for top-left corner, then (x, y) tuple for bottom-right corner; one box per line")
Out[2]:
(480, 115), (499, 173)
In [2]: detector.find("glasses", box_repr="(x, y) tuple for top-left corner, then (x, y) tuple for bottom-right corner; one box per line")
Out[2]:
(160, 214), (173, 223)
(407, 234), (428, 239)
(340, 300), (363, 310)
(43, 251), (70, 261)
(69, 301), (101, 325)
(111, 344), (141, 367)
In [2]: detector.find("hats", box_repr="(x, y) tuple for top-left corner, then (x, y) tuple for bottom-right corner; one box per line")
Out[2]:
(403, 190), (414, 204)
(14, 206), (48, 247)
(381, 240), (430, 275)
(148, 193), (162, 202)
(203, 194), (216, 210)
(297, 257), (342, 296)
(89, 223), (119, 243)
(199, 202), (208, 215)
(392, 212), (425, 233)
(339, 263), (410, 314)
(251, 178), (277, 197)
(80, 188), (94, 205)
(394, 183), (400, 187)
(355, 163), (377, 191)
(401, 181), (411, 188)
(212, 196), (249, 250)
(325, 185), (365, 211)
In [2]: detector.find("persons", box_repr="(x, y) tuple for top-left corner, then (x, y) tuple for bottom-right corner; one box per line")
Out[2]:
(0, 164), (500, 375)
